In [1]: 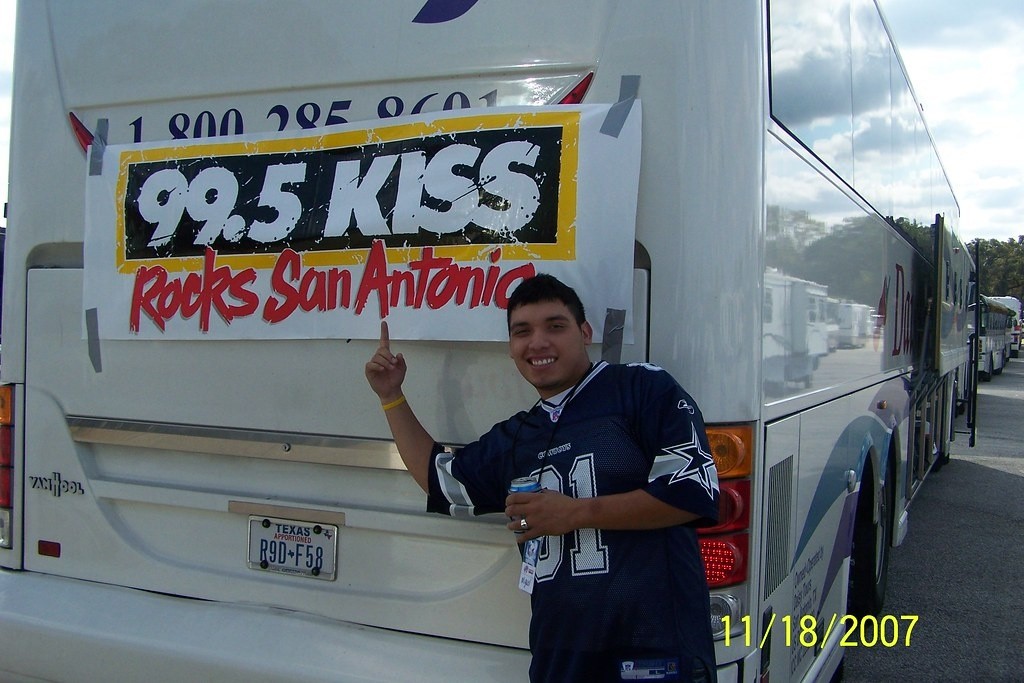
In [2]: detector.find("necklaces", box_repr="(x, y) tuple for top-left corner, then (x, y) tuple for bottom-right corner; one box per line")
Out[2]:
(541, 361), (594, 423)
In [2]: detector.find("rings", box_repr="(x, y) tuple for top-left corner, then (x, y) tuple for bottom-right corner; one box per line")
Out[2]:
(521, 515), (529, 530)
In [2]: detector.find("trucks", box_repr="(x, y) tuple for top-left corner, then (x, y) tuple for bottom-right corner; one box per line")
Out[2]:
(763, 266), (875, 398)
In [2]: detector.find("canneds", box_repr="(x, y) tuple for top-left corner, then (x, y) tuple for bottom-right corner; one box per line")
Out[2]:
(509, 477), (542, 540)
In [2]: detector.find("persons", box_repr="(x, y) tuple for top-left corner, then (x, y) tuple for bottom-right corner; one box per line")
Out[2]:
(365, 272), (720, 683)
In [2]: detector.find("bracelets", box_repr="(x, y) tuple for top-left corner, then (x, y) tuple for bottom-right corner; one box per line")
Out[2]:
(382, 394), (406, 413)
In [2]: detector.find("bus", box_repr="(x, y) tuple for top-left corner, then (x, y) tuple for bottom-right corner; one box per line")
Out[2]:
(2, 1), (976, 683)
(966, 294), (1023, 382)
